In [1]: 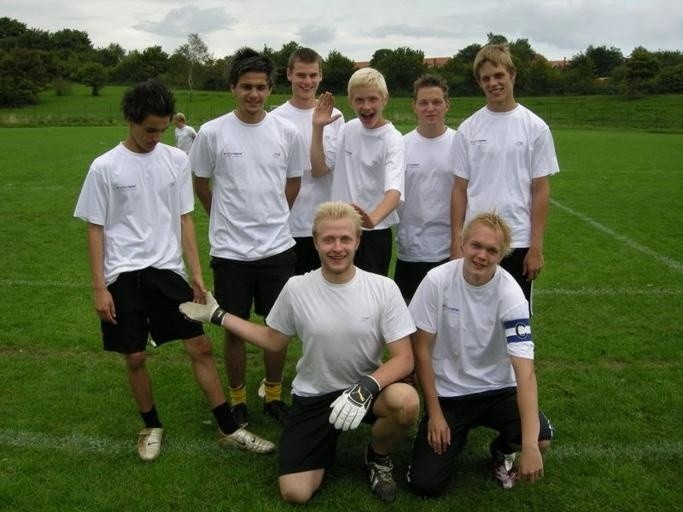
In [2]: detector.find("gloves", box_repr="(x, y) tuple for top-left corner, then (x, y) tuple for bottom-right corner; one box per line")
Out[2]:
(329, 374), (381, 432)
(178, 290), (229, 328)
(489, 438), (519, 490)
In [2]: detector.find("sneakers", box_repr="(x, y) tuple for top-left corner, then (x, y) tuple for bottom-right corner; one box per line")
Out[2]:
(364, 441), (396, 504)
(217, 422), (275, 454)
(263, 395), (291, 427)
(231, 402), (247, 427)
(137, 427), (163, 462)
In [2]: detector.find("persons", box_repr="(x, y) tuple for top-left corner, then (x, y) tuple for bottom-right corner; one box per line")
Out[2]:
(404, 210), (557, 499)
(393, 73), (462, 390)
(306, 62), (406, 280)
(176, 198), (426, 505)
(262, 47), (346, 276)
(70, 78), (278, 461)
(442, 43), (564, 318)
(172, 110), (200, 157)
(185, 52), (304, 428)
